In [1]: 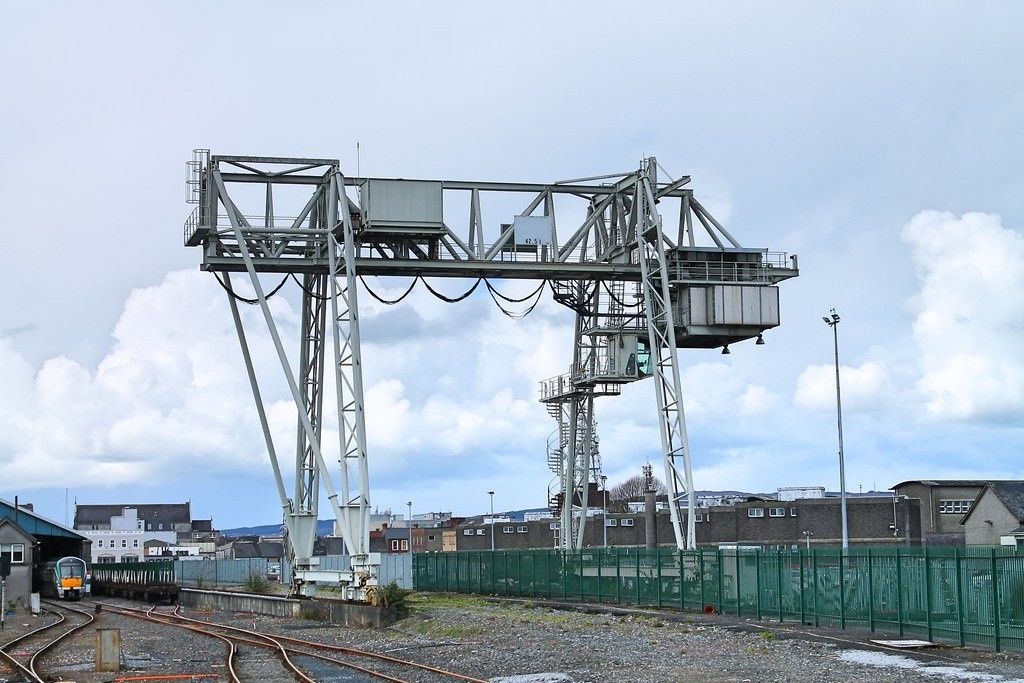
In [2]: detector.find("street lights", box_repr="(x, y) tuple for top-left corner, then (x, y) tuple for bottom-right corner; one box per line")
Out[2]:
(406, 502), (413, 555)
(487, 491), (495, 551)
(822, 306), (850, 566)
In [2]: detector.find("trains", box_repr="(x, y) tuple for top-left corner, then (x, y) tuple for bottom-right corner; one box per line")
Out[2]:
(33, 556), (87, 601)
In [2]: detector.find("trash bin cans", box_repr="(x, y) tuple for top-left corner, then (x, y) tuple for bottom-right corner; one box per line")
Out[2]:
(28, 590), (41, 616)
